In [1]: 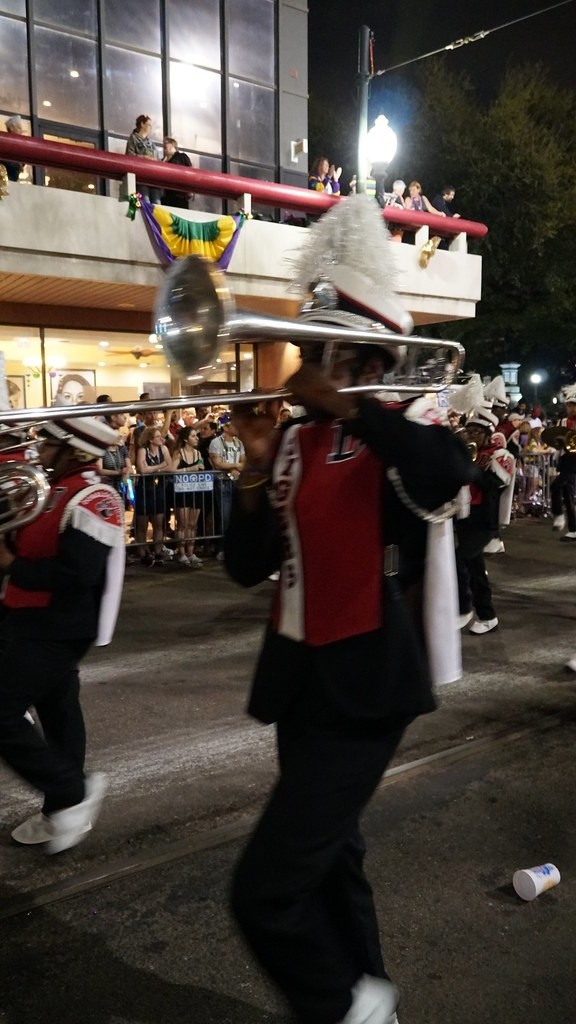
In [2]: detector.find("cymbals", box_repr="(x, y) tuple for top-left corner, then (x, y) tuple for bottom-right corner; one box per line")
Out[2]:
(540, 425), (576, 450)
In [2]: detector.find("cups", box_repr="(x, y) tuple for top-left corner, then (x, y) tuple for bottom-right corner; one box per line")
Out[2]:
(513, 860), (560, 900)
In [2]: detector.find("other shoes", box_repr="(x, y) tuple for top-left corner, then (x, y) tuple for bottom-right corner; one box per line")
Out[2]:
(189, 554), (204, 567)
(127, 556), (135, 563)
(162, 546), (174, 556)
(141, 556), (155, 567)
(10, 813), (55, 844)
(46, 779), (106, 855)
(483, 538), (505, 552)
(342, 978), (398, 1024)
(216, 552), (223, 560)
(177, 556), (191, 566)
(565, 532), (576, 538)
(458, 612), (474, 629)
(469, 617), (498, 634)
(552, 515), (566, 530)
(156, 552), (173, 560)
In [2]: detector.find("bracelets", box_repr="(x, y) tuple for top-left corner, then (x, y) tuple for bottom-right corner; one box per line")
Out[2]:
(183, 468), (187, 471)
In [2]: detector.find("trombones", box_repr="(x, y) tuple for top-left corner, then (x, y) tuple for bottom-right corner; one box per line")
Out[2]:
(454, 426), (478, 463)
(1, 253), (471, 431)
(1, 420), (52, 454)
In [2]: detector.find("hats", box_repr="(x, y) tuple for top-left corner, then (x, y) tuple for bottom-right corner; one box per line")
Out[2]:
(33, 415), (122, 457)
(564, 392), (575, 404)
(289, 193), (414, 350)
(218, 414), (234, 429)
(464, 407), (499, 434)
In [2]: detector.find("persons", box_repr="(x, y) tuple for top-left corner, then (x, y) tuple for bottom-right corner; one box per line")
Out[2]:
(0, 394), (128, 855)
(6, 379), (22, 410)
(54, 375), (95, 406)
(0, 115), (27, 181)
(126, 113), (193, 210)
(223, 191), (471, 1024)
(275, 402), (308, 428)
(309, 158), (462, 251)
(98, 394), (246, 571)
(376, 372), (576, 637)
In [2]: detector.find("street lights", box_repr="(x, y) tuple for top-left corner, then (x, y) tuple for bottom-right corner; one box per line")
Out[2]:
(367, 114), (398, 208)
(531, 373), (542, 404)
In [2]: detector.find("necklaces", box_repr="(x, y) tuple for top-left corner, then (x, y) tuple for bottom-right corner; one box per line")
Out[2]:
(219, 436), (237, 464)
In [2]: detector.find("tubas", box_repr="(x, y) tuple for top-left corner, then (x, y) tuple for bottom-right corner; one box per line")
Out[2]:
(0, 456), (55, 535)
